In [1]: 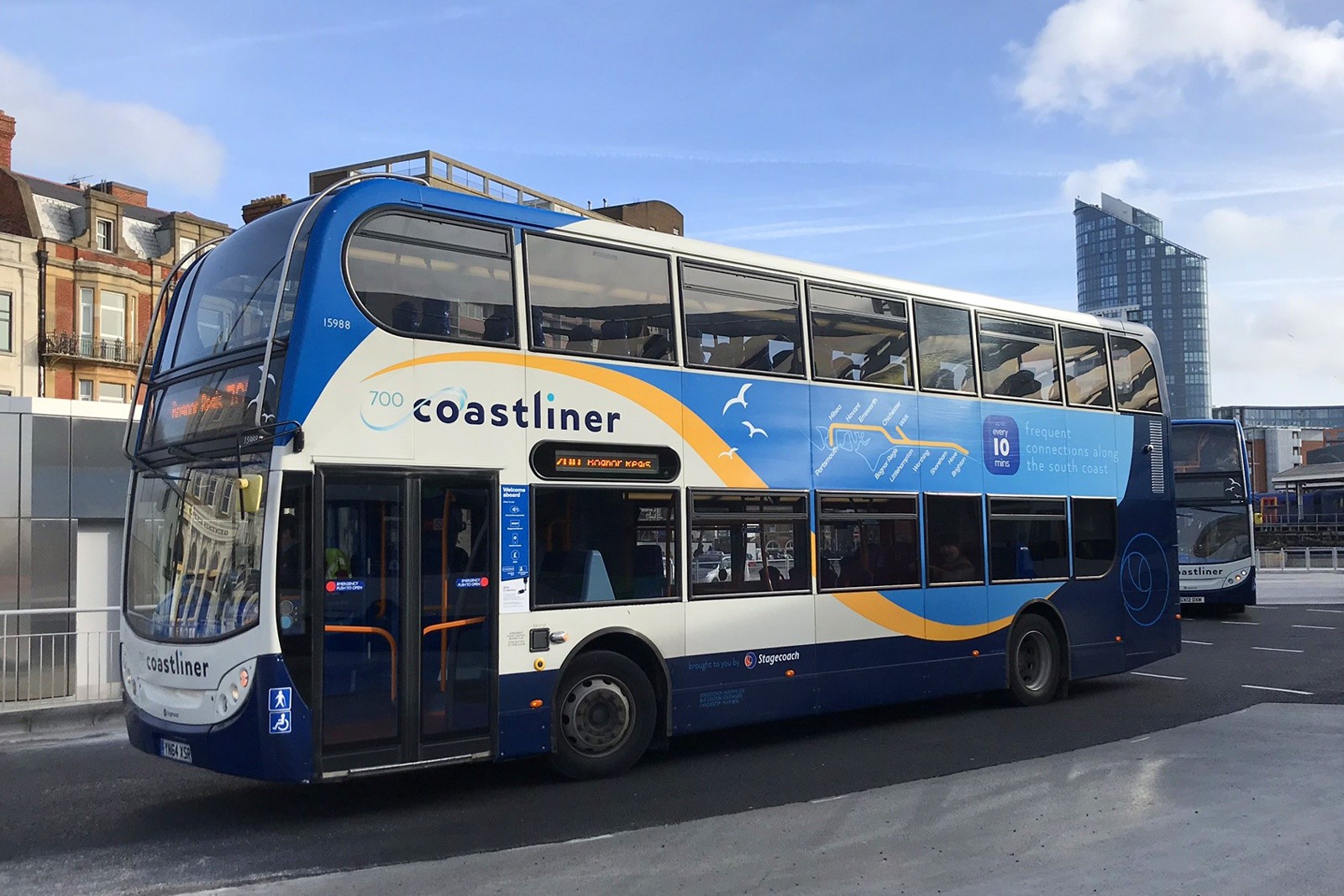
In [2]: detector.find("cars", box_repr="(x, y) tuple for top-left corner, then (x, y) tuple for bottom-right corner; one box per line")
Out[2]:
(662, 557), (675, 581)
(691, 554), (751, 584)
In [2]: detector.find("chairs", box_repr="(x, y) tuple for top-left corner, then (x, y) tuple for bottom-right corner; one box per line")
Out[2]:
(629, 532), (1081, 599)
(365, 286), (1122, 407)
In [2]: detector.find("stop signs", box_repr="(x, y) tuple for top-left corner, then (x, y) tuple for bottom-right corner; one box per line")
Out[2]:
(853, 532), (859, 538)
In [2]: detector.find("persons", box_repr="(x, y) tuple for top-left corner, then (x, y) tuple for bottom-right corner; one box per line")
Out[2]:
(930, 538), (975, 583)
(277, 521), (326, 591)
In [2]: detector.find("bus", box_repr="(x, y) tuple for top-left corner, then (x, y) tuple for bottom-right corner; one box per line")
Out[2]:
(118, 172), (1182, 785)
(1170, 417), (1262, 612)
